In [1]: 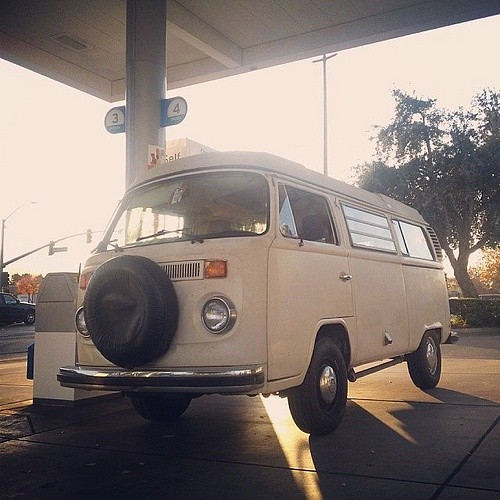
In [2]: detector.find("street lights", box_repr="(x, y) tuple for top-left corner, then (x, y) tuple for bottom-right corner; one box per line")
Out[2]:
(0, 200), (37, 293)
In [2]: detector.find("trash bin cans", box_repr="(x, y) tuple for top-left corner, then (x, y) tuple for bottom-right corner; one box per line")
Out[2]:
(27, 272), (123, 408)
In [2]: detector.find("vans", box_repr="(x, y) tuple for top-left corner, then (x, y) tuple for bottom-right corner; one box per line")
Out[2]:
(57, 148), (460, 437)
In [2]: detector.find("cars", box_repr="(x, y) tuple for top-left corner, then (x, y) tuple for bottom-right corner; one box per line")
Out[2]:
(0, 291), (35, 327)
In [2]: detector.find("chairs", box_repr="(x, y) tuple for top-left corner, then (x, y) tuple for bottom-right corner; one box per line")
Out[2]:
(303, 215), (324, 240)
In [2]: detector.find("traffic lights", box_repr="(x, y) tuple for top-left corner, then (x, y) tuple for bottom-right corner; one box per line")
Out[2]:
(87, 229), (92, 243)
